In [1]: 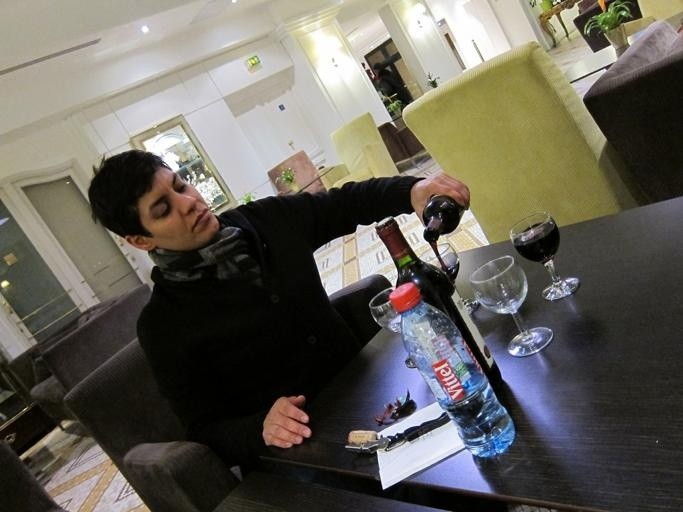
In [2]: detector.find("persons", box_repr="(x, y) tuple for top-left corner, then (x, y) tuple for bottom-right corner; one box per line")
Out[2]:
(83, 147), (472, 479)
(361, 62), (414, 118)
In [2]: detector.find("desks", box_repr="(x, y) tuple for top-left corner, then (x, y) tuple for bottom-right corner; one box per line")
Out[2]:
(564, 12), (683, 83)
(538, 0), (583, 48)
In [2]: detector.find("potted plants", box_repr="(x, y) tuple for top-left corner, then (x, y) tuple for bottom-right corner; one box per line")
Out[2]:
(584, 0), (636, 59)
(386, 100), (403, 117)
(529, 0), (557, 11)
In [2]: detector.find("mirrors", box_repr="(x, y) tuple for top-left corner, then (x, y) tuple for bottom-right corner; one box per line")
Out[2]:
(131, 114), (236, 227)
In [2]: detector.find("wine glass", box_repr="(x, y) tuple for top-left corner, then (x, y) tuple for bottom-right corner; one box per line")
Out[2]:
(417, 242), (481, 317)
(468, 255), (553, 358)
(509, 208), (580, 301)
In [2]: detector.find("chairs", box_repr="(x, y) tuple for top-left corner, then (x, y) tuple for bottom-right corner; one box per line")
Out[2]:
(0, 439), (70, 512)
(267, 151), (327, 199)
(401, 43), (638, 245)
(0, 284), (151, 422)
(62, 273), (392, 512)
(330, 113), (400, 189)
(377, 122), (425, 163)
(582, 11), (682, 201)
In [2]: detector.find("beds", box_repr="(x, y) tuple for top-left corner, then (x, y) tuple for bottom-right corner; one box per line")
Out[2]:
(266, 197), (683, 512)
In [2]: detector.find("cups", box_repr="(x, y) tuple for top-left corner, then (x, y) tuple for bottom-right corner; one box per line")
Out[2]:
(367, 286), (402, 336)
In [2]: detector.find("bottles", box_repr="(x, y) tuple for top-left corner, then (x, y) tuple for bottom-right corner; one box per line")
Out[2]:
(422, 194), (465, 242)
(374, 216), (502, 390)
(388, 281), (517, 459)
